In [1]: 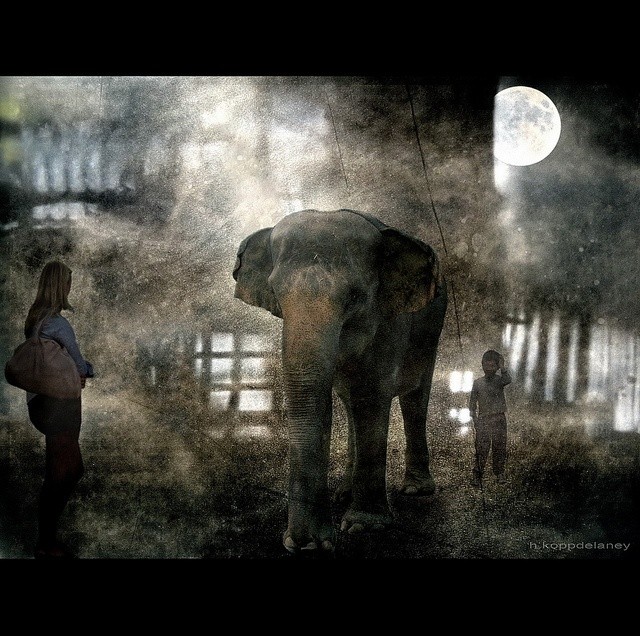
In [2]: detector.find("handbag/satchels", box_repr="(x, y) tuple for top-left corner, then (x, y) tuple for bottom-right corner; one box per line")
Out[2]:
(4, 318), (81, 400)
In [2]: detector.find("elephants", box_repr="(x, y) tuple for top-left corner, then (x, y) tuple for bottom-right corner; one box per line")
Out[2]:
(233, 210), (447, 555)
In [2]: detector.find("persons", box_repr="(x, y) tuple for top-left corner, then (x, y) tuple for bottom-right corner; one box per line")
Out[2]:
(471, 352), (509, 486)
(25, 259), (95, 483)
